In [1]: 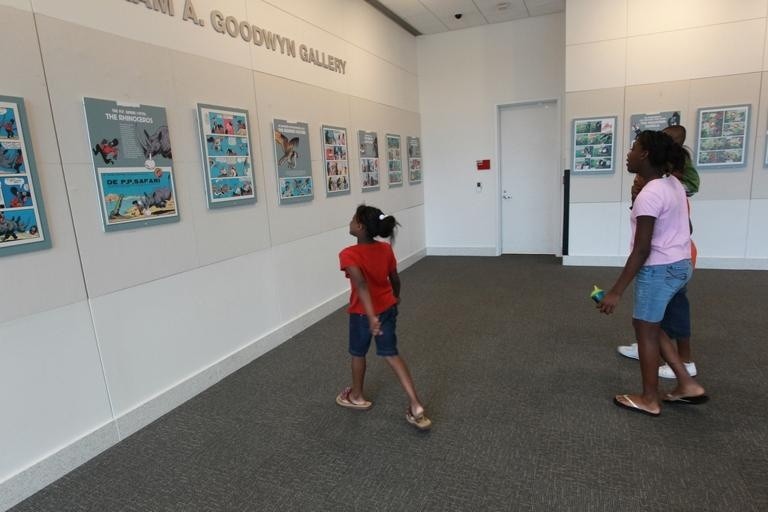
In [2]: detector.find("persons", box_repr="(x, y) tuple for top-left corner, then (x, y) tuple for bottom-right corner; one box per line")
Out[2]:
(596, 130), (710, 416)
(336, 203), (433, 431)
(618, 124), (700, 380)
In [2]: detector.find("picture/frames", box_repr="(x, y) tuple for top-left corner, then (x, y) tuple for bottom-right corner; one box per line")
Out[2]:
(196, 102), (257, 210)
(693, 103), (752, 170)
(385, 133), (403, 188)
(320, 124), (351, 197)
(570, 116), (618, 175)
(0, 94), (52, 257)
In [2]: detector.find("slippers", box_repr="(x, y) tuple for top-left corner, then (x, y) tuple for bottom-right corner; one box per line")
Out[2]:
(614, 393), (660, 416)
(663, 392), (710, 405)
(337, 387), (372, 409)
(406, 406), (432, 431)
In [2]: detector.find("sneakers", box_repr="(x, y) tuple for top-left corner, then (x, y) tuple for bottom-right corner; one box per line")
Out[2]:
(659, 361), (698, 378)
(618, 342), (639, 359)
